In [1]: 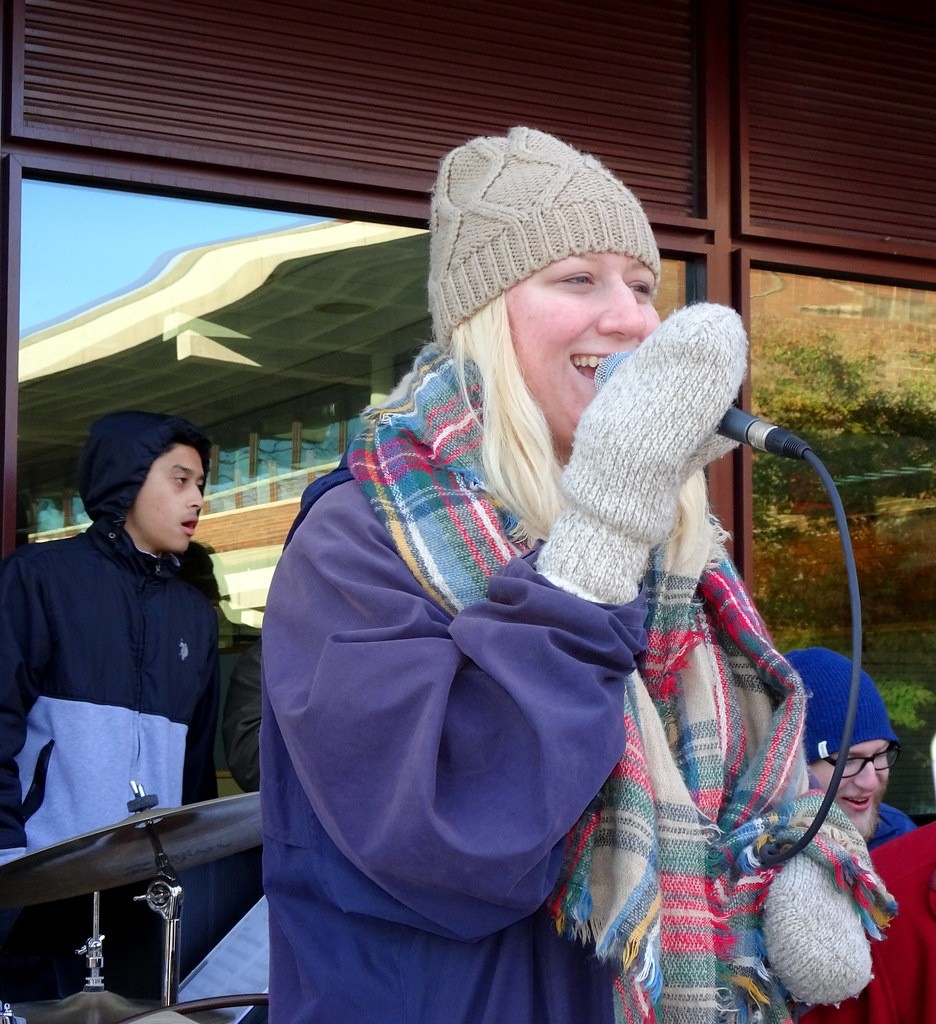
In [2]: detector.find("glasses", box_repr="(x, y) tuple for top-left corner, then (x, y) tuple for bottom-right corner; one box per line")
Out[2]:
(824, 744), (903, 778)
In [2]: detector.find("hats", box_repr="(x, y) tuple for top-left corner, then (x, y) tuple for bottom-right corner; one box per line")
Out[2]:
(782, 648), (899, 766)
(427, 127), (661, 353)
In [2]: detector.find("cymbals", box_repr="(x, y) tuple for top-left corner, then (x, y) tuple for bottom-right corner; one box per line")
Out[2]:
(3, 992), (234, 1023)
(0, 790), (265, 918)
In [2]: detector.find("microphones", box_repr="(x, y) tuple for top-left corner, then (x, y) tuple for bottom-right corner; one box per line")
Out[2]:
(595, 351), (812, 461)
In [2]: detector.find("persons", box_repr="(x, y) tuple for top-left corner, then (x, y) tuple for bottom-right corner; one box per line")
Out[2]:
(0, 408), (266, 1009)
(777, 649), (935, 1024)
(256, 124), (873, 1024)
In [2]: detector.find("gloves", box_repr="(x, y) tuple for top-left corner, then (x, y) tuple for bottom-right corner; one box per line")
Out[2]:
(536, 302), (747, 604)
(764, 846), (873, 1005)
